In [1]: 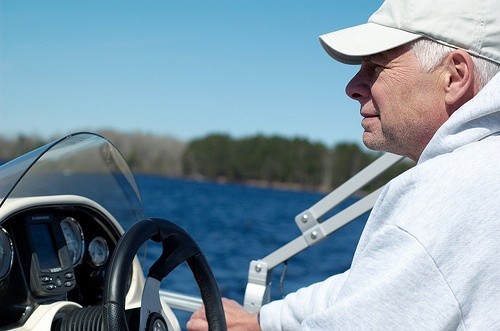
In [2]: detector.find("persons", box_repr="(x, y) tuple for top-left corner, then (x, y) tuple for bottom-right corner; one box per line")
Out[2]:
(186, 1), (499, 331)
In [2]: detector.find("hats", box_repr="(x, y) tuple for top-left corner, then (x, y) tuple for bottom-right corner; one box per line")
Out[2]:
(319, 0), (500, 65)
